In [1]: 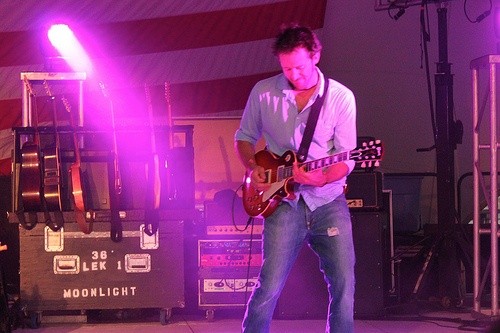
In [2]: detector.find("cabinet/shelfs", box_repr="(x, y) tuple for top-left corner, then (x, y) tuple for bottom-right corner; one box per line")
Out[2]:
(471, 54), (500, 323)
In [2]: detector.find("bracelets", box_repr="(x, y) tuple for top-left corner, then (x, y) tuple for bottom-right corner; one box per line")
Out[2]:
(323, 171), (329, 184)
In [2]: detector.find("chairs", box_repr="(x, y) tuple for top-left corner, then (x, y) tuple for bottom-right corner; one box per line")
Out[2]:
(411, 171), (500, 306)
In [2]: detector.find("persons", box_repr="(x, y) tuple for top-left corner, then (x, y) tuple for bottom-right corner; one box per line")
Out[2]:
(231, 25), (359, 333)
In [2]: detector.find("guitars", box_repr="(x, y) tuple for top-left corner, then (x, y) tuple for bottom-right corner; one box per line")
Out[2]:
(20, 74), (178, 210)
(241, 140), (383, 219)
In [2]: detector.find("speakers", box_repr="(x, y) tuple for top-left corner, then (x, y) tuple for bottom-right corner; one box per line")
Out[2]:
(303, 210), (387, 319)
